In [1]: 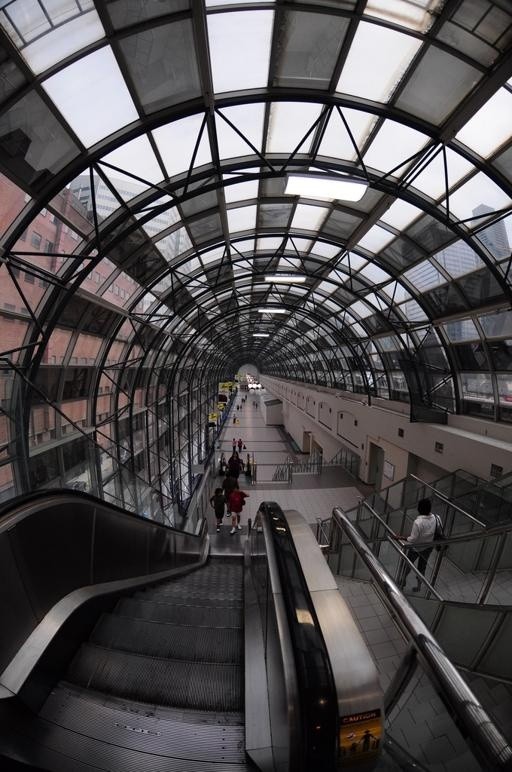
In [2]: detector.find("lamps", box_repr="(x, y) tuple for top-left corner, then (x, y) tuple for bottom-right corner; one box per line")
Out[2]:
(264, 275), (306, 284)
(282, 172), (370, 203)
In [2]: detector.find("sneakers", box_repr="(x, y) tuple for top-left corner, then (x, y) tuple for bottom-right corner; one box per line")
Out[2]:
(237, 523), (242, 530)
(230, 527), (236, 534)
(216, 526), (221, 531)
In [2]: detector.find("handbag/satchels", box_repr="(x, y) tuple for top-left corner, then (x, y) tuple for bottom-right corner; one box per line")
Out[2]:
(433, 513), (450, 552)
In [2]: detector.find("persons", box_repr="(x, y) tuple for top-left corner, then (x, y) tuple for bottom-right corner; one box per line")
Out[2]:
(394, 499), (443, 592)
(210, 438), (251, 534)
